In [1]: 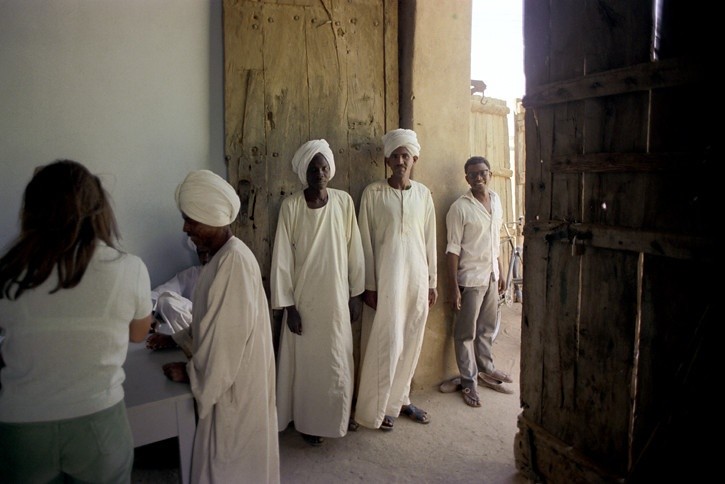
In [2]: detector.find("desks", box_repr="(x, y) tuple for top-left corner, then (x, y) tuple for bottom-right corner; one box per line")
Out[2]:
(123, 321), (197, 484)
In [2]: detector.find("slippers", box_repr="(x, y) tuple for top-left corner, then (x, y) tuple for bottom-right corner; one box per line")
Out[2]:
(460, 388), (481, 407)
(488, 369), (513, 383)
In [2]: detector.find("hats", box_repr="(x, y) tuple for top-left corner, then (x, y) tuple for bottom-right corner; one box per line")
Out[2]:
(380, 129), (420, 158)
(174, 170), (241, 227)
(291, 139), (336, 184)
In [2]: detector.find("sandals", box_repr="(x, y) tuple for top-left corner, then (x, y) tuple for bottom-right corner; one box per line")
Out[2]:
(400, 404), (431, 423)
(381, 415), (394, 429)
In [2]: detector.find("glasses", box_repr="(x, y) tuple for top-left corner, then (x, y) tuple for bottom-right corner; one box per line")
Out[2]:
(466, 169), (492, 178)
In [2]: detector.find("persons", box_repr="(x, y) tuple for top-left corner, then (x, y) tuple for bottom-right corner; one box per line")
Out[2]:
(151, 169), (280, 483)
(445, 156), (517, 408)
(0, 160), (153, 484)
(359, 128), (439, 430)
(271, 138), (367, 447)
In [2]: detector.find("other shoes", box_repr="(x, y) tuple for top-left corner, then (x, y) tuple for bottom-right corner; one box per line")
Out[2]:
(348, 418), (360, 431)
(302, 434), (325, 446)
(476, 372), (514, 394)
(440, 376), (462, 393)
